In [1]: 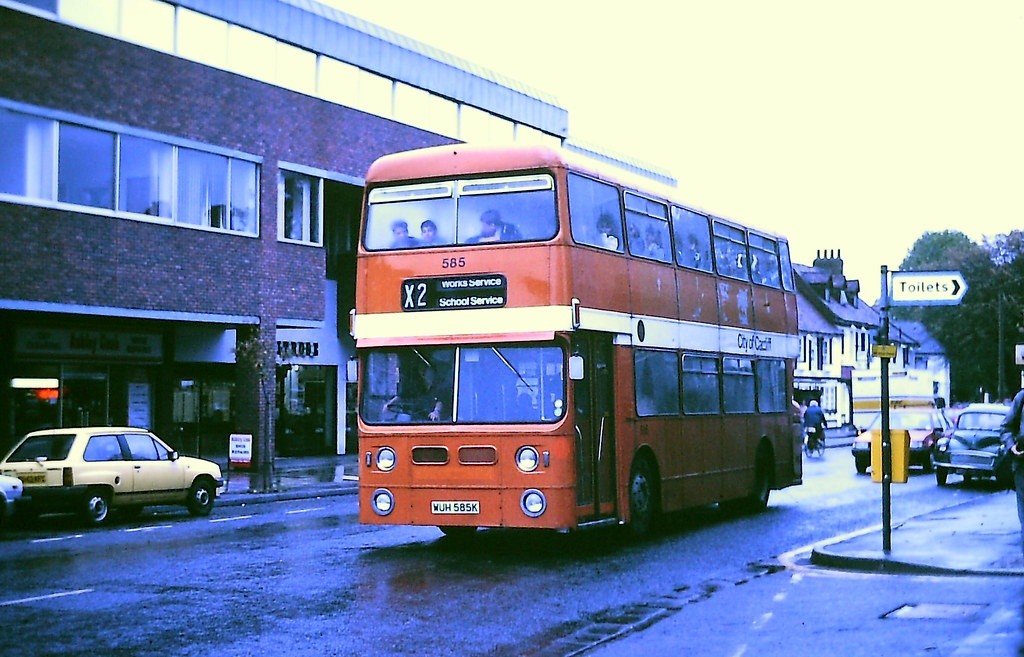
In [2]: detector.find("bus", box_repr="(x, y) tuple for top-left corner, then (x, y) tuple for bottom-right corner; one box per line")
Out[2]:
(333, 140), (805, 547)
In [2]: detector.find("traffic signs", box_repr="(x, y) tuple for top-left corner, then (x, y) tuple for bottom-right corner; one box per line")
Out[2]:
(885, 268), (970, 306)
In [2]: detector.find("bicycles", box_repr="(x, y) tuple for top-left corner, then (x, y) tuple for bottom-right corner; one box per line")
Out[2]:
(800, 423), (827, 457)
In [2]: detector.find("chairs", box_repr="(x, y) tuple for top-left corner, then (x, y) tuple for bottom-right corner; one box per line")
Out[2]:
(576, 223), (794, 296)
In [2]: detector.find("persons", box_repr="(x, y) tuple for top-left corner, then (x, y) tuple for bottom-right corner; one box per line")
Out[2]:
(388, 210), (764, 281)
(383, 363), (561, 421)
(999, 390), (1024, 537)
(797, 399), (828, 451)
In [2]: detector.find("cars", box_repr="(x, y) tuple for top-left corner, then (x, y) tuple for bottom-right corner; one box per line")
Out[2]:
(944, 399), (974, 423)
(0, 425), (227, 523)
(0, 470), (24, 523)
(852, 405), (955, 473)
(932, 402), (1020, 487)
